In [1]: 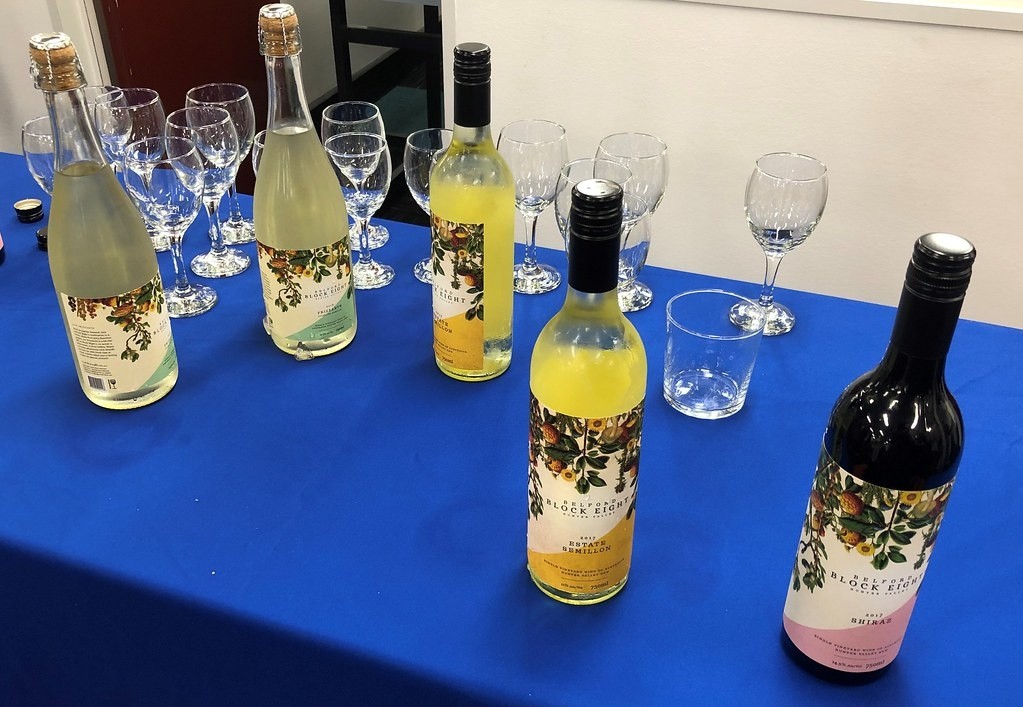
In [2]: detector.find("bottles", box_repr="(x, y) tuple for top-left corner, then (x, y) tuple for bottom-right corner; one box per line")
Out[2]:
(431, 42), (514, 382)
(29, 31), (179, 410)
(780, 230), (977, 689)
(527, 178), (648, 607)
(253, 4), (358, 357)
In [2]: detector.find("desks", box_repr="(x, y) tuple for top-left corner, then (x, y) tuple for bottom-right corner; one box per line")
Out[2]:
(0, 151), (1023, 707)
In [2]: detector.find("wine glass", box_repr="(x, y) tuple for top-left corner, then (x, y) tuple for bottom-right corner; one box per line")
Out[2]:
(592, 133), (670, 312)
(617, 192), (653, 289)
(93, 88), (171, 252)
(123, 135), (217, 318)
(496, 120), (569, 295)
(728, 150), (828, 337)
(320, 101), (395, 253)
(183, 82), (257, 246)
(404, 128), (454, 286)
(164, 107), (251, 279)
(324, 131), (396, 290)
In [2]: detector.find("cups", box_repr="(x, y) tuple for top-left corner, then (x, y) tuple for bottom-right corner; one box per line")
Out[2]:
(82, 84), (133, 165)
(22, 116), (56, 197)
(253, 130), (267, 177)
(555, 158), (634, 242)
(662, 289), (768, 420)
